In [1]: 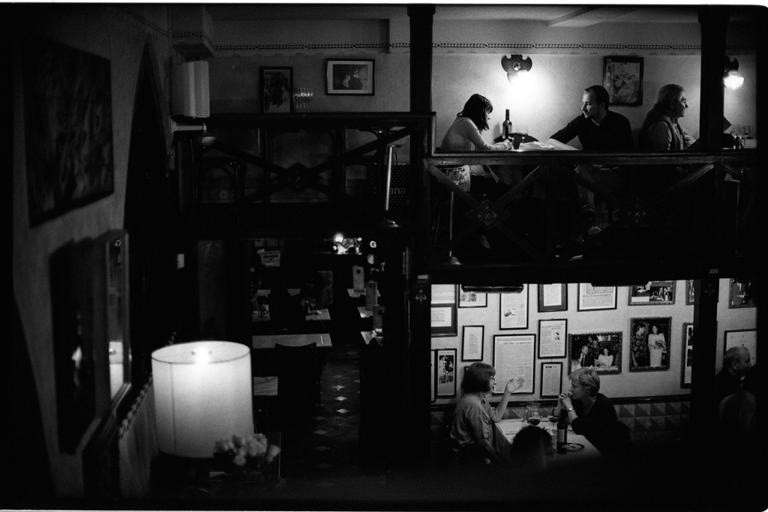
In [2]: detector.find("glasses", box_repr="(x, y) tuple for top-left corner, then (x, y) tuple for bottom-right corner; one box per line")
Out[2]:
(147, 340), (257, 467)
(719, 56), (744, 91)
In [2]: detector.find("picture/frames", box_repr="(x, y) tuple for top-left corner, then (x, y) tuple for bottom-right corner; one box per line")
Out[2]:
(724, 329), (756, 366)
(568, 331), (622, 375)
(628, 280), (676, 306)
(458, 284), (487, 308)
(680, 322), (693, 389)
(577, 282), (617, 312)
(538, 283), (568, 313)
(685, 280), (695, 305)
(434, 348), (456, 399)
(499, 283), (529, 330)
(323, 57), (375, 97)
(629, 317), (671, 372)
(728, 278), (756, 309)
(538, 319), (568, 359)
(461, 325), (485, 362)
(601, 55), (644, 108)
(491, 334), (536, 396)
(431, 284), (457, 305)
(258, 66), (295, 115)
(431, 305), (459, 337)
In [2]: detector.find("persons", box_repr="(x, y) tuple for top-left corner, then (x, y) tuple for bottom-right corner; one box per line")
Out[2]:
(450, 362), (524, 487)
(441, 94), (509, 200)
(594, 348), (614, 367)
(551, 366), (631, 450)
(639, 84), (687, 198)
(550, 85), (635, 218)
(632, 323), (648, 367)
(577, 345), (595, 367)
(648, 325), (667, 368)
(715, 345), (751, 398)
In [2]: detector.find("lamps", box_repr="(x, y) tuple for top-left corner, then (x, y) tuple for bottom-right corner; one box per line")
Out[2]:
(719, 56), (744, 91)
(147, 340), (257, 467)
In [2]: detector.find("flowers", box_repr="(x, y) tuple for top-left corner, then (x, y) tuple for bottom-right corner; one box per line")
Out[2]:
(205, 434), (290, 473)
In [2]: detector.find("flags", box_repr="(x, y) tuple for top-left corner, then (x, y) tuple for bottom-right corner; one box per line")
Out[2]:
(208, 475), (286, 499)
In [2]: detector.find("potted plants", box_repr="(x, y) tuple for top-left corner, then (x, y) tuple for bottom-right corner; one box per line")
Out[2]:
(601, 55), (644, 108)
(258, 66), (295, 115)
(323, 57), (375, 97)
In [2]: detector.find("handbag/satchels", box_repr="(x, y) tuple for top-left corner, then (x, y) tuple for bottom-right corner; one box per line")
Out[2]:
(567, 408), (574, 412)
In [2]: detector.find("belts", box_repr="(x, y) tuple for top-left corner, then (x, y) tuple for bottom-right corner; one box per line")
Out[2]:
(528, 408), (540, 426)
(730, 124), (751, 149)
(548, 406), (559, 433)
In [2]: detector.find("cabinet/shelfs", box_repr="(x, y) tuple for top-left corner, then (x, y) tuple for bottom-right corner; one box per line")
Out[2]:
(556, 407), (568, 454)
(502, 111), (512, 142)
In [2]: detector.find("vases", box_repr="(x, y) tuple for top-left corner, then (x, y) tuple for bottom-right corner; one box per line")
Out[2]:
(205, 434), (290, 473)
(208, 475), (286, 499)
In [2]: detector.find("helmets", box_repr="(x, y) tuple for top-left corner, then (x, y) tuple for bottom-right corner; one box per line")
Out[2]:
(567, 443), (585, 452)
